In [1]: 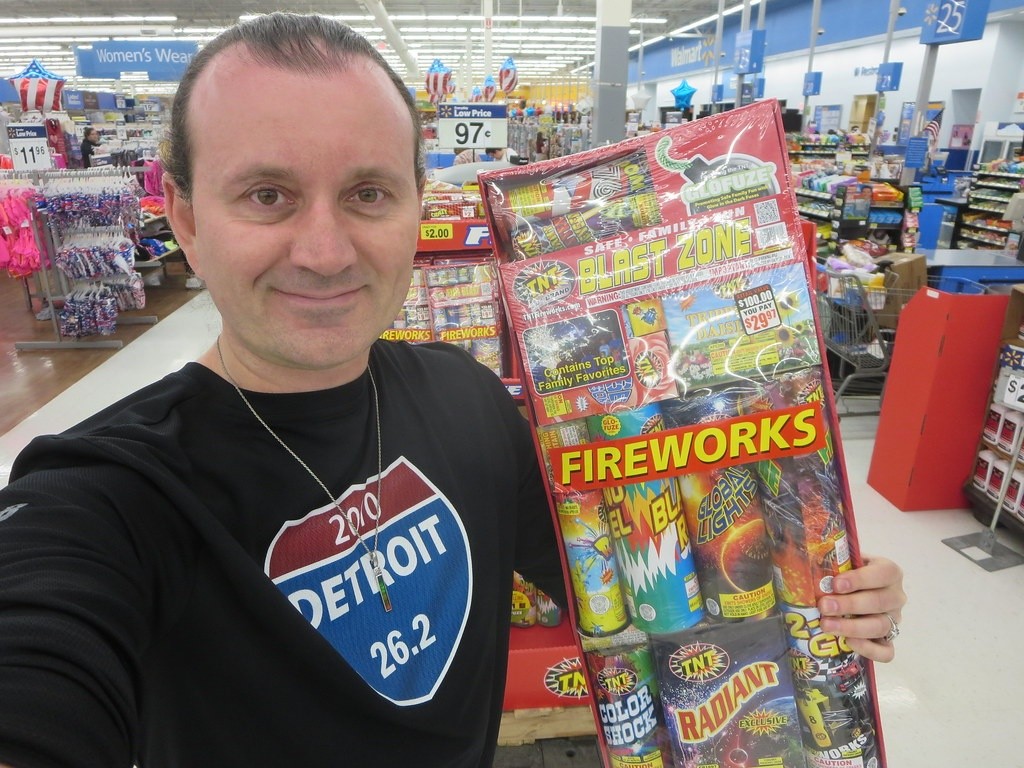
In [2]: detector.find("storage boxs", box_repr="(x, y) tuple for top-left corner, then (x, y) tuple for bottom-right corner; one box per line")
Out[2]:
(874, 252), (928, 330)
(369, 252), (505, 379)
(476, 97), (887, 767)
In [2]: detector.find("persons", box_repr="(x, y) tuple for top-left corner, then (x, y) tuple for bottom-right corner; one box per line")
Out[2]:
(0, 15), (899, 768)
(534, 131), (548, 162)
(81, 125), (101, 169)
(451, 147), (482, 166)
(485, 147), (519, 163)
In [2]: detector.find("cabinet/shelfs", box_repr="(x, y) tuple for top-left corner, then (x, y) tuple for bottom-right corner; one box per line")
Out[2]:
(133, 208), (192, 272)
(949, 163), (1024, 250)
(0, 165), (159, 350)
(961, 284), (1024, 537)
(786, 133), (927, 271)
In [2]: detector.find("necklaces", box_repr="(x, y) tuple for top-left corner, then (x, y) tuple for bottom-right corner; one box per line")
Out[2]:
(210, 332), (396, 613)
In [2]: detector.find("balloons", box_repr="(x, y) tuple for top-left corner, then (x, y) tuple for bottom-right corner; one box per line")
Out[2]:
(508, 96), (593, 119)
(5, 57), (68, 113)
(631, 90), (652, 111)
(425, 53), (519, 105)
(670, 78), (698, 109)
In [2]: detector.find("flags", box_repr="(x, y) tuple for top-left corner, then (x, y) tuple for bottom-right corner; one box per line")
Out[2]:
(924, 109), (943, 142)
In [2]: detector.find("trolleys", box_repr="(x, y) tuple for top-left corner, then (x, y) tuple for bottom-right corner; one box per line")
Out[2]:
(812, 256), (990, 423)
